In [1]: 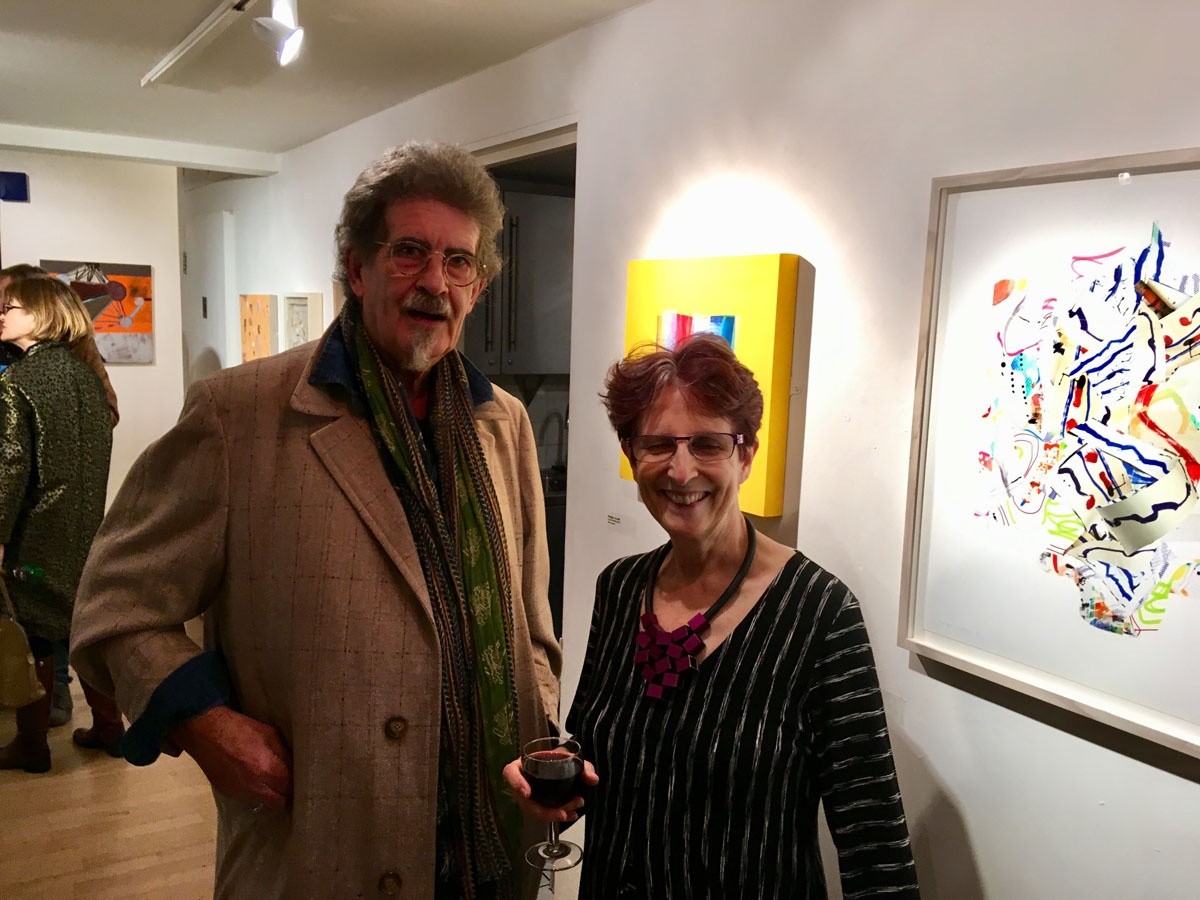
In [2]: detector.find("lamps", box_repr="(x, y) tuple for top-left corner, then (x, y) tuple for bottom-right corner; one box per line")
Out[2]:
(254, 0), (304, 67)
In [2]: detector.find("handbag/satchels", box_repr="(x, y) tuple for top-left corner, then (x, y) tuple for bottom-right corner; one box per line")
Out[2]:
(1, 575), (47, 708)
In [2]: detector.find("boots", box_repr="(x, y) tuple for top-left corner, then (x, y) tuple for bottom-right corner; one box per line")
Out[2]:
(73, 673), (125, 758)
(0, 654), (55, 773)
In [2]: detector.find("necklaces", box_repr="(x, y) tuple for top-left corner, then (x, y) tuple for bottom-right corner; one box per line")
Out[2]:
(634, 513), (757, 700)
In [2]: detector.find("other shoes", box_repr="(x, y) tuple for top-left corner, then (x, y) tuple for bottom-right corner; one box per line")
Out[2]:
(49, 682), (73, 728)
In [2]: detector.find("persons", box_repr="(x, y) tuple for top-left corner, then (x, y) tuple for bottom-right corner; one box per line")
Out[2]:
(70, 139), (563, 900)
(501, 334), (926, 899)
(0, 261), (129, 775)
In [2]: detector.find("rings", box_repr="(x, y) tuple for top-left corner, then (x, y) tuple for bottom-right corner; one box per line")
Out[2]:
(252, 802), (264, 814)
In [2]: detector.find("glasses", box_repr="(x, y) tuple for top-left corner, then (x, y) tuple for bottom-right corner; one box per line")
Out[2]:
(625, 433), (743, 463)
(3, 303), (23, 315)
(372, 241), (488, 287)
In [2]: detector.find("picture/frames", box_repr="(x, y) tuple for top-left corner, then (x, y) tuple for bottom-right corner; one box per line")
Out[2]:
(894, 149), (1200, 763)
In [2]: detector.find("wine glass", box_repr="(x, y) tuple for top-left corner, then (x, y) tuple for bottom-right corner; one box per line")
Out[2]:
(520, 738), (583, 870)
